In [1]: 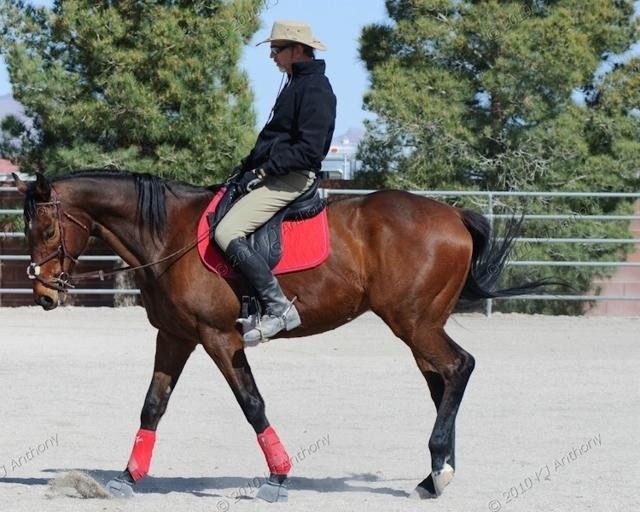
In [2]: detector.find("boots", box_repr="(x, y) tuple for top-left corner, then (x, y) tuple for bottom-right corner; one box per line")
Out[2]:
(225, 236), (301, 341)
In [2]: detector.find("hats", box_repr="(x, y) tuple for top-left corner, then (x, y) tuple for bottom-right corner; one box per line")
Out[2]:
(256, 20), (327, 50)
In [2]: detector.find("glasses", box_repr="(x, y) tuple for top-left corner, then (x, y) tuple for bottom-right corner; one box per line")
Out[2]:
(270, 44), (289, 55)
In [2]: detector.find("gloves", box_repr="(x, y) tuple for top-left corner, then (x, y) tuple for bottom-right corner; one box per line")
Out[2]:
(227, 165), (267, 193)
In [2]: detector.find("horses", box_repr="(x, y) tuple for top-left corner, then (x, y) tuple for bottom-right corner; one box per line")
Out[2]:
(22, 164), (574, 505)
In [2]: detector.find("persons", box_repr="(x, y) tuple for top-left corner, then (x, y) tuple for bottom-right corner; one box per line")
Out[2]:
(212, 20), (337, 344)
